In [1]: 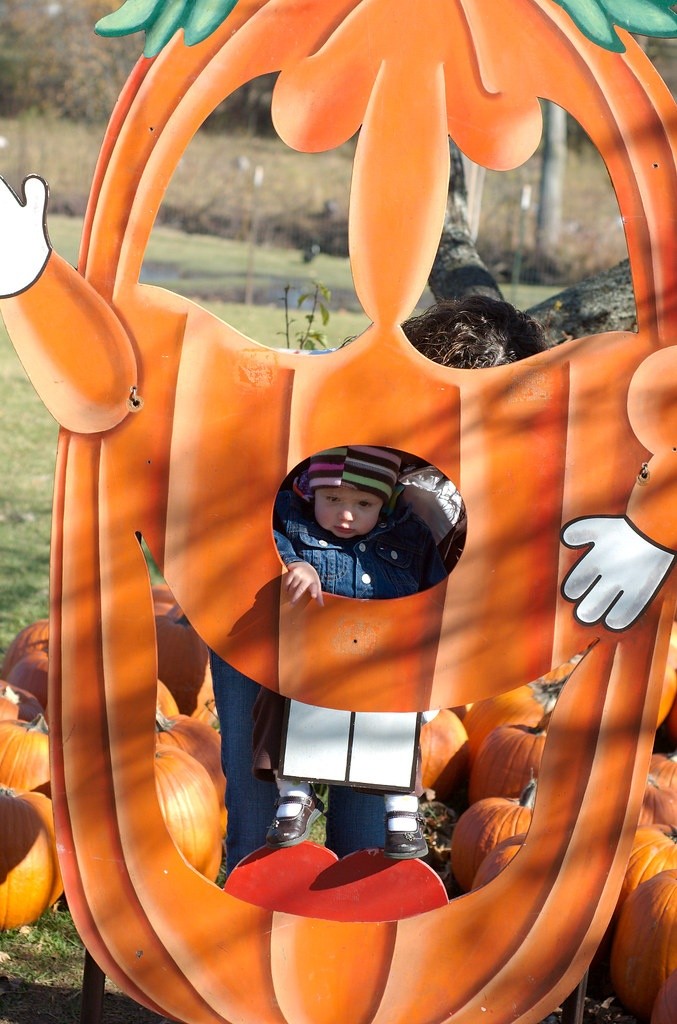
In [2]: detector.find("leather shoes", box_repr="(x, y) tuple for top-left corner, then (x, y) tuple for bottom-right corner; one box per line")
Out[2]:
(384, 811), (429, 859)
(266, 783), (324, 848)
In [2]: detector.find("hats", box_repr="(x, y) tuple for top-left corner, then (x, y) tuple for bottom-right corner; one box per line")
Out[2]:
(308, 446), (401, 502)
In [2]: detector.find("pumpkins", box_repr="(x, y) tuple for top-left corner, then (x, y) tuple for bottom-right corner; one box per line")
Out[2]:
(0, 585), (676, 1023)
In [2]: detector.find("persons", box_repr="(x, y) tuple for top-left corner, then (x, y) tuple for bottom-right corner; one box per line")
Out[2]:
(252, 444), (455, 861)
(209, 295), (548, 877)
